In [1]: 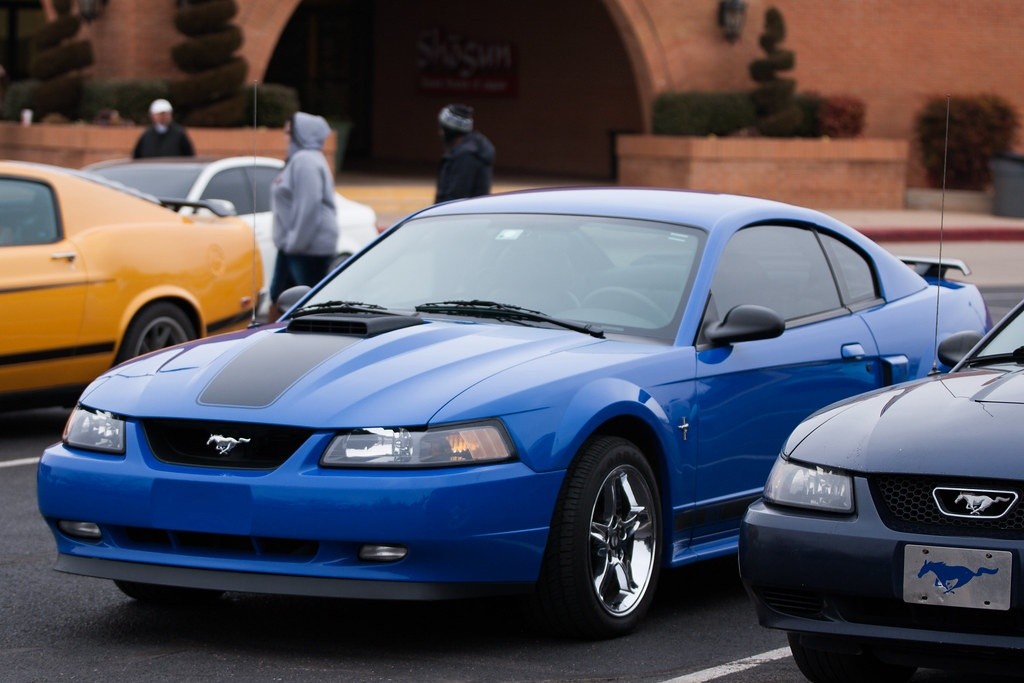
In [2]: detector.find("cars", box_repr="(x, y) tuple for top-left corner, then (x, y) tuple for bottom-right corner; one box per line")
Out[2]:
(738, 300), (1023, 682)
(0, 160), (264, 412)
(78, 154), (390, 329)
(34, 187), (994, 645)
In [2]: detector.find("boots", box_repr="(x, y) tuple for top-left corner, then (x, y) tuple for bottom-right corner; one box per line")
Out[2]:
(264, 302), (284, 324)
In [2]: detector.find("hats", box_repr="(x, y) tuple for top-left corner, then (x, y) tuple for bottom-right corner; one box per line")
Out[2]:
(439, 104), (473, 131)
(150, 99), (172, 112)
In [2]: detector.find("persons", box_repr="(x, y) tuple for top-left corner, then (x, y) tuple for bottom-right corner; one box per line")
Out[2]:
(434, 104), (494, 203)
(267, 112), (338, 323)
(131, 99), (194, 159)
